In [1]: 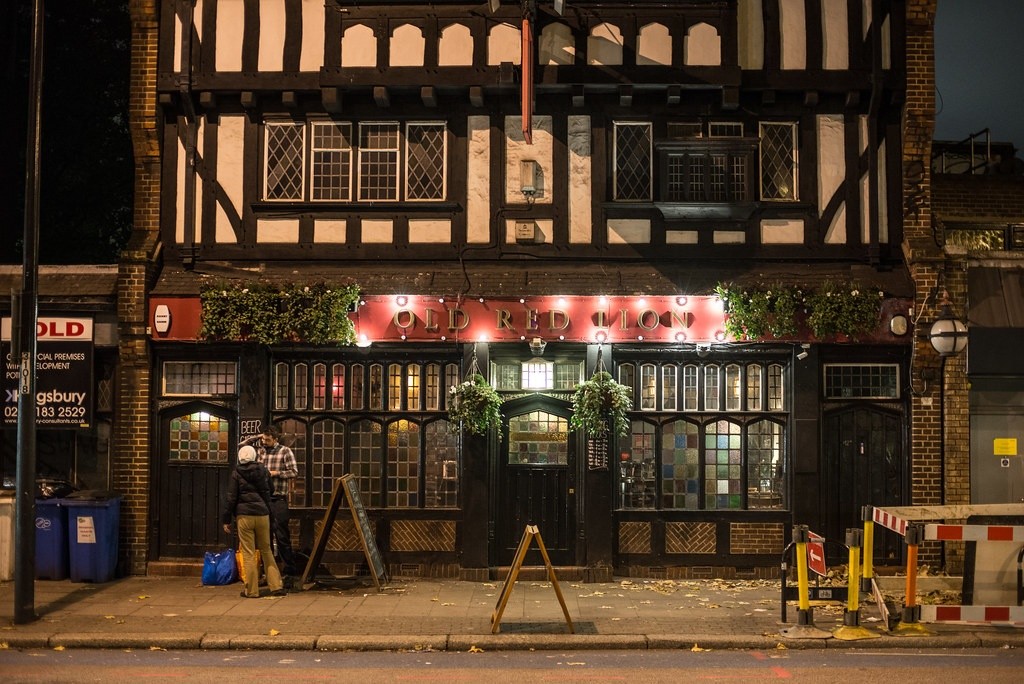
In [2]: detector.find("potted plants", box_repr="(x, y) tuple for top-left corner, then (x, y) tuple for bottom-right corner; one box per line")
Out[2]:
(566, 370), (634, 438)
(447, 374), (509, 444)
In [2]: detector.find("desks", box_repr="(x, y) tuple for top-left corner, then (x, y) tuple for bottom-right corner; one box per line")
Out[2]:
(749, 495), (781, 507)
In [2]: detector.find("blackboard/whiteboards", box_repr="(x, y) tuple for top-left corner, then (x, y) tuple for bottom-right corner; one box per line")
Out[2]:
(494, 525), (571, 623)
(301, 474), (387, 580)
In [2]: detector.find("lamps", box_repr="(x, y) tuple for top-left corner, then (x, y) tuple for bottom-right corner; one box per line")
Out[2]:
(927, 273), (969, 357)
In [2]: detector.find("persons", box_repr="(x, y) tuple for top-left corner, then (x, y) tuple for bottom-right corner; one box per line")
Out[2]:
(237, 426), (298, 589)
(223, 445), (285, 598)
(619, 447), (641, 506)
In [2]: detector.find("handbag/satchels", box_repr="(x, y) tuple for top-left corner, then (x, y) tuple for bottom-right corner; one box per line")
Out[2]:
(235, 541), (262, 586)
(201, 549), (237, 586)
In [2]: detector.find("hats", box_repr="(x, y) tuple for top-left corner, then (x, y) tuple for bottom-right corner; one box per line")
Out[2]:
(238, 445), (256, 464)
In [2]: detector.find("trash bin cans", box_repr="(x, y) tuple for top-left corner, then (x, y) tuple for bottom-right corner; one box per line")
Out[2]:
(34, 480), (69, 580)
(62, 489), (120, 585)
(0, 476), (18, 582)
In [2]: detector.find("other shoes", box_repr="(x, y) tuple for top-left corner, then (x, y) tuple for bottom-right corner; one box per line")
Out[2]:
(283, 575), (293, 589)
(240, 591), (248, 597)
(272, 588), (286, 596)
(258, 575), (267, 586)
